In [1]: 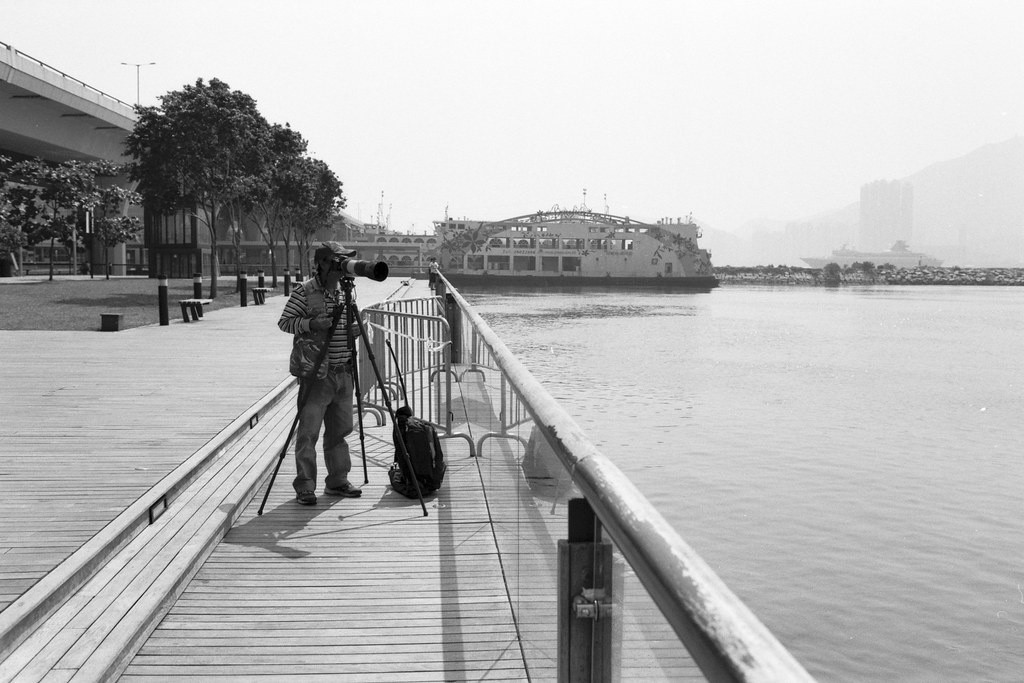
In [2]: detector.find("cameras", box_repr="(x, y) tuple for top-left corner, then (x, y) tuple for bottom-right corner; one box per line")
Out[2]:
(330, 255), (389, 282)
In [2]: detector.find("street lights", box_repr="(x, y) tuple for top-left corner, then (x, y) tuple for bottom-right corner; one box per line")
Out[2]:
(121, 63), (156, 107)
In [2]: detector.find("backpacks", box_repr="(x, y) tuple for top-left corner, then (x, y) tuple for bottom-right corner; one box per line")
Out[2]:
(388, 407), (446, 500)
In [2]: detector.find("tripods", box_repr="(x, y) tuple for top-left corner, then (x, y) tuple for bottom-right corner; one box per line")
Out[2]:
(257, 282), (429, 517)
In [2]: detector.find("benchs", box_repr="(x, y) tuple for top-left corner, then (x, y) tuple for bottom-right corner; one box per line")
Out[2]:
(178, 299), (214, 323)
(251, 287), (273, 305)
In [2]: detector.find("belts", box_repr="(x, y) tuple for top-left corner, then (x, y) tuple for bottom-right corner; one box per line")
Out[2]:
(328, 364), (351, 373)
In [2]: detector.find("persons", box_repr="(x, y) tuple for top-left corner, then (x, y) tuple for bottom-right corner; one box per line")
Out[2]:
(278, 241), (367, 505)
(80, 260), (91, 274)
(428, 257), (440, 290)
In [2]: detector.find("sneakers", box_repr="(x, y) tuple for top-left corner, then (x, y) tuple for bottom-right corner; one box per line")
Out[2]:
(324, 482), (362, 497)
(296, 490), (317, 505)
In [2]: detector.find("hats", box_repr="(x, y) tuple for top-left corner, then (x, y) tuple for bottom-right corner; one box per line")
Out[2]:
(314, 240), (356, 265)
